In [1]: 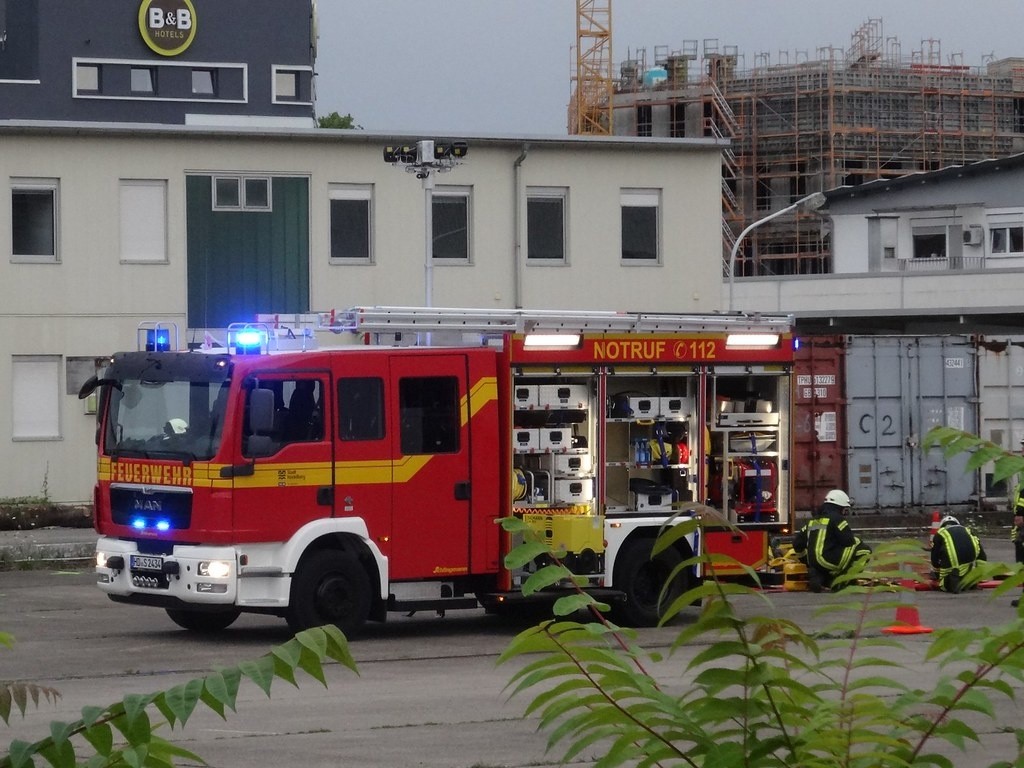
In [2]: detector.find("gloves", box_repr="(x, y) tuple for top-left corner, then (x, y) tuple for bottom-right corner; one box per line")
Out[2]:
(798, 555), (808, 564)
(929, 570), (940, 580)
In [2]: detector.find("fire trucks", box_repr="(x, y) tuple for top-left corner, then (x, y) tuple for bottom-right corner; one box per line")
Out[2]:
(77, 305), (798, 641)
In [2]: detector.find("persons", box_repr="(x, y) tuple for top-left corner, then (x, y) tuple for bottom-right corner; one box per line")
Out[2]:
(163, 418), (186, 436)
(1010, 437), (1024, 607)
(792, 489), (871, 593)
(925, 516), (987, 593)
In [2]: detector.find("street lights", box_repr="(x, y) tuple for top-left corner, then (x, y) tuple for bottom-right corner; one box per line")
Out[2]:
(729, 191), (827, 311)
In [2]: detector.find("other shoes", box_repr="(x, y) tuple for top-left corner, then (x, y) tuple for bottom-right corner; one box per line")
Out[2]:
(808, 568), (821, 593)
(833, 572), (845, 592)
(969, 581), (977, 590)
(951, 568), (961, 593)
(1011, 599), (1019, 608)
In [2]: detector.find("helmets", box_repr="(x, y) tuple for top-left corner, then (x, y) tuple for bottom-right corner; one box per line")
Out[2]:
(939, 515), (960, 527)
(824, 490), (856, 507)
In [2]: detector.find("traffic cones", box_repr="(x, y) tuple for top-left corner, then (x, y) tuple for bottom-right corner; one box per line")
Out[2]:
(880, 567), (934, 634)
(922, 510), (939, 550)
